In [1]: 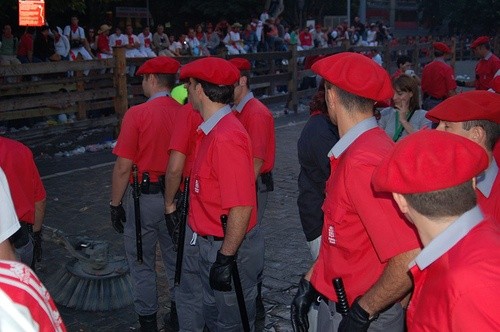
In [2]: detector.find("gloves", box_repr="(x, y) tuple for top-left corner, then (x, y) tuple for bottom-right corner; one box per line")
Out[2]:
(32, 232), (41, 268)
(165, 210), (179, 242)
(210, 250), (237, 291)
(109, 200), (126, 233)
(291, 278), (316, 332)
(337, 300), (370, 332)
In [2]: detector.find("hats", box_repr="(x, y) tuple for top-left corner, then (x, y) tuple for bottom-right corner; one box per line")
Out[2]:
(135, 56), (181, 75)
(229, 58), (250, 70)
(425, 91), (500, 123)
(231, 23), (243, 28)
(470, 36), (487, 48)
(374, 131), (488, 191)
(180, 57), (240, 85)
(431, 42), (451, 53)
(312, 53), (393, 100)
(98, 25), (112, 33)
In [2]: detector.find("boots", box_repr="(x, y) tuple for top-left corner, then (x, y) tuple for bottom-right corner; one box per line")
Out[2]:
(138, 313), (157, 332)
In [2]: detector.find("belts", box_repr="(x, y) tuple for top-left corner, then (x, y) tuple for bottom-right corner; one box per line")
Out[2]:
(200, 236), (225, 241)
(312, 290), (345, 314)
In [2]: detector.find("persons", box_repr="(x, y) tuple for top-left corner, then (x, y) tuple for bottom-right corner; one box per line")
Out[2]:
(0, 0), (499, 332)
(292, 52), (423, 332)
(370, 127), (500, 332)
(174, 58), (266, 332)
